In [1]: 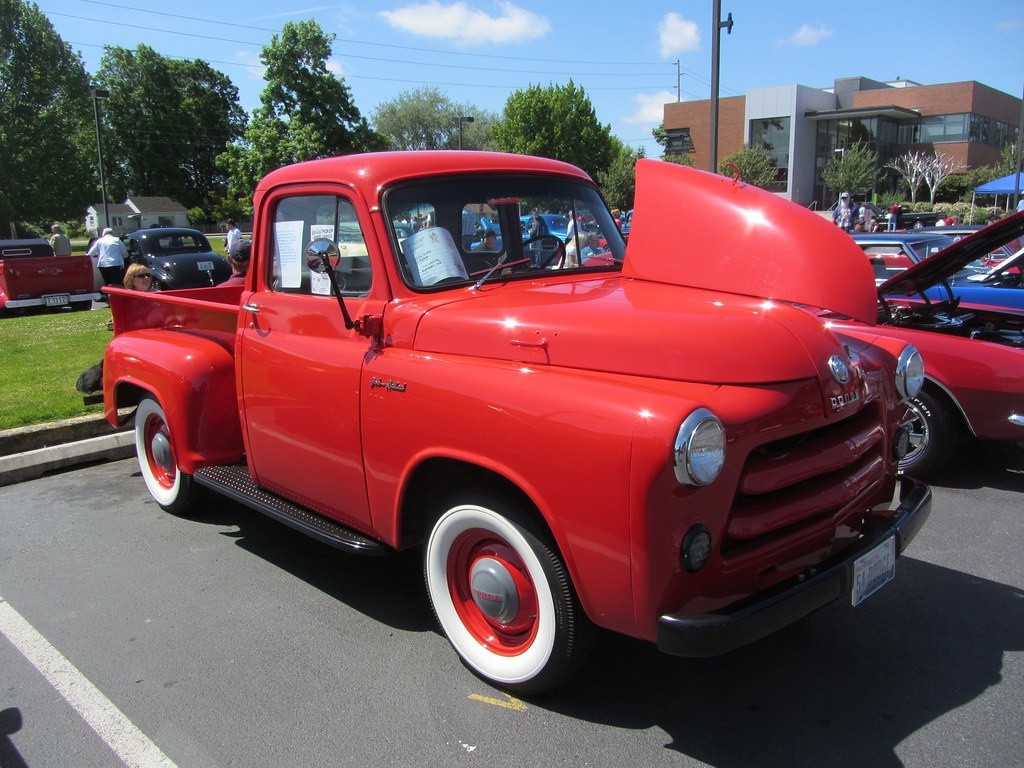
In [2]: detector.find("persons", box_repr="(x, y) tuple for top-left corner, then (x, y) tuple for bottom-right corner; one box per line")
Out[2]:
(473, 230), (497, 253)
(888, 202), (900, 232)
(526, 208), (549, 267)
(50, 225), (71, 257)
(988, 209), (1001, 221)
(1016, 195), (1024, 212)
(217, 240), (252, 286)
(832, 192), (859, 233)
(462, 209), (476, 251)
(935, 215), (958, 227)
(224, 219), (241, 254)
(123, 262), (161, 292)
(395, 211), (435, 233)
(559, 209), (633, 268)
(88, 228), (129, 287)
(854, 205), (880, 233)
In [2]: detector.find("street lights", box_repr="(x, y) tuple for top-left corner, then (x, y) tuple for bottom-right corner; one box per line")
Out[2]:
(90, 89), (111, 230)
(451, 115), (475, 151)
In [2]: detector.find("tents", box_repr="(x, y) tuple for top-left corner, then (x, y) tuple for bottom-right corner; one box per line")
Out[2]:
(969, 172), (1024, 226)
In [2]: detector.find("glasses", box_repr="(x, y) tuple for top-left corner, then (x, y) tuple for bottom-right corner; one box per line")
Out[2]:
(590, 237), (597, 241)
(134, 272), (150, 278)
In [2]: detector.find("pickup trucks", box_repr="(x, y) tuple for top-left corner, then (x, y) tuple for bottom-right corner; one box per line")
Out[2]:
(74, 148), (935, 700)
(0, 238), (102, 317)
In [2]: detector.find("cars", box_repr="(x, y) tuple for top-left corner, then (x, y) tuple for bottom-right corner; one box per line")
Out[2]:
(796, 206), (1024, 480)
(330, 201), (1023, 312)
(86, 226), (233, 292)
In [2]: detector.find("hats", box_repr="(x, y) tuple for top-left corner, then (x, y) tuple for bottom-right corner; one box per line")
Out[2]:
(841, 193), (849, 199)
(224, 219), (235, 225)
(228, 240), (251, 262)
(532, 207), (540, 212)
